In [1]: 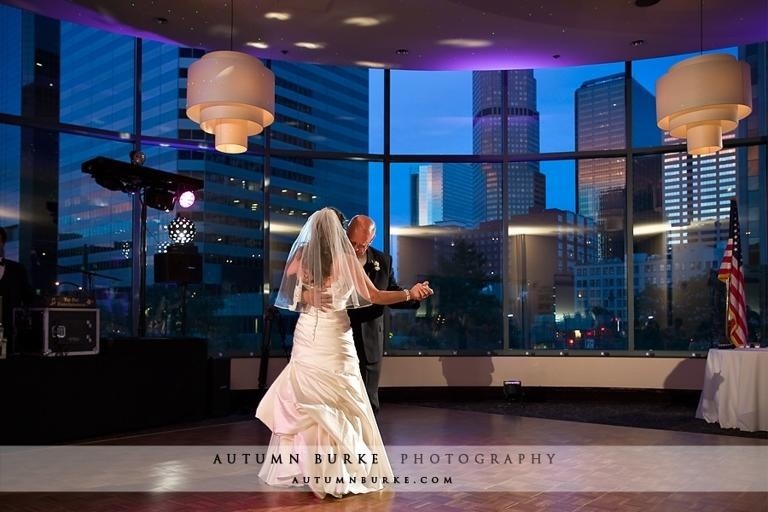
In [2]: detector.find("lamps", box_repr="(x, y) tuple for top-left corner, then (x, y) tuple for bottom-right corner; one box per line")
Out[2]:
(186, 1), (276, 154)
(655, 1), (753, 155)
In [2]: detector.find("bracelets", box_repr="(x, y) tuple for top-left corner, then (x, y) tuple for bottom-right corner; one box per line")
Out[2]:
(403, 287), (411, 303)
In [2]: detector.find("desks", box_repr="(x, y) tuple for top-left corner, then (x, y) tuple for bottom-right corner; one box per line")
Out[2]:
(709, 347), (768, 432)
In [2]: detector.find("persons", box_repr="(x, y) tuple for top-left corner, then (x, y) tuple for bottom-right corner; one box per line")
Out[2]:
(293, 214), (435, 418)
(252, 206), (435, 499)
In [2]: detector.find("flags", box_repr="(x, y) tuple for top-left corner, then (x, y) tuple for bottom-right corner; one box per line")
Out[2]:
(717, 197), (750, 347)
